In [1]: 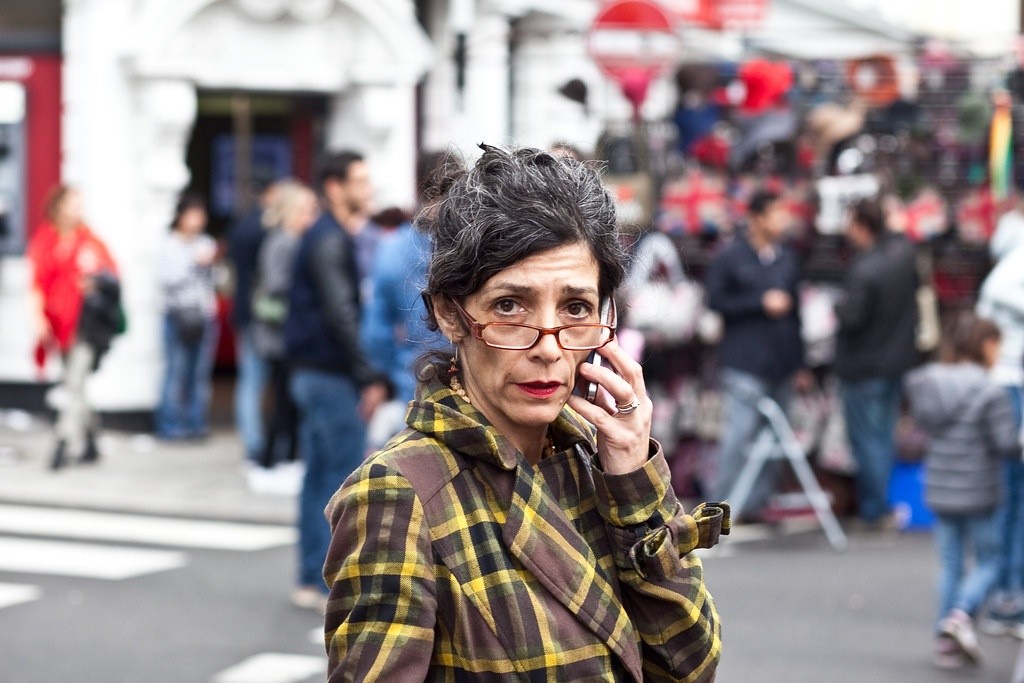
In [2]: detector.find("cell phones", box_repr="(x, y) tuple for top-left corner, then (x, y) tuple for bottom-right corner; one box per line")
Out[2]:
(586, 299), (611, 403)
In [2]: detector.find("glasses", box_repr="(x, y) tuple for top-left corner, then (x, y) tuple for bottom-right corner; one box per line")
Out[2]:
(443, 292), (618, 350)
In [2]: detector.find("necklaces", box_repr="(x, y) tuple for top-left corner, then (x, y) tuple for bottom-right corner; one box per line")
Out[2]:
(449, 374), (471, 401)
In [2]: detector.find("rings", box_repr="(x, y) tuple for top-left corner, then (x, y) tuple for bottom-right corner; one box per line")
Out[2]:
(619, 398), (644, 416)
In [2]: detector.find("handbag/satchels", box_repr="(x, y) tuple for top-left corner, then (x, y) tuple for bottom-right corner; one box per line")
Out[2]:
(615, 229), (700, 344)
(913, 284), (938, 349)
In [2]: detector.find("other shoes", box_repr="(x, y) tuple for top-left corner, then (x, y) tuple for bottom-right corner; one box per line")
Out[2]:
(936, 612), (982, 663)
(76, 430), (101, 462)
(984, 604), (1024, 639)
(932, 638), (970, 670)
(290, 576), (330, 611)
(49, 439), (68, 471)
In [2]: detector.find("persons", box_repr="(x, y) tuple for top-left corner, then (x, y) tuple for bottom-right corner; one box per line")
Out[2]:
(704, 192), (803, 518)
(835, 204), (921, 528)
(326, 146), (735, 683)
(156, 196), (232, 439)
(237, 150), (449, 604)
(27, 188), (128, 465)
(906, 179), (1023, 668)
